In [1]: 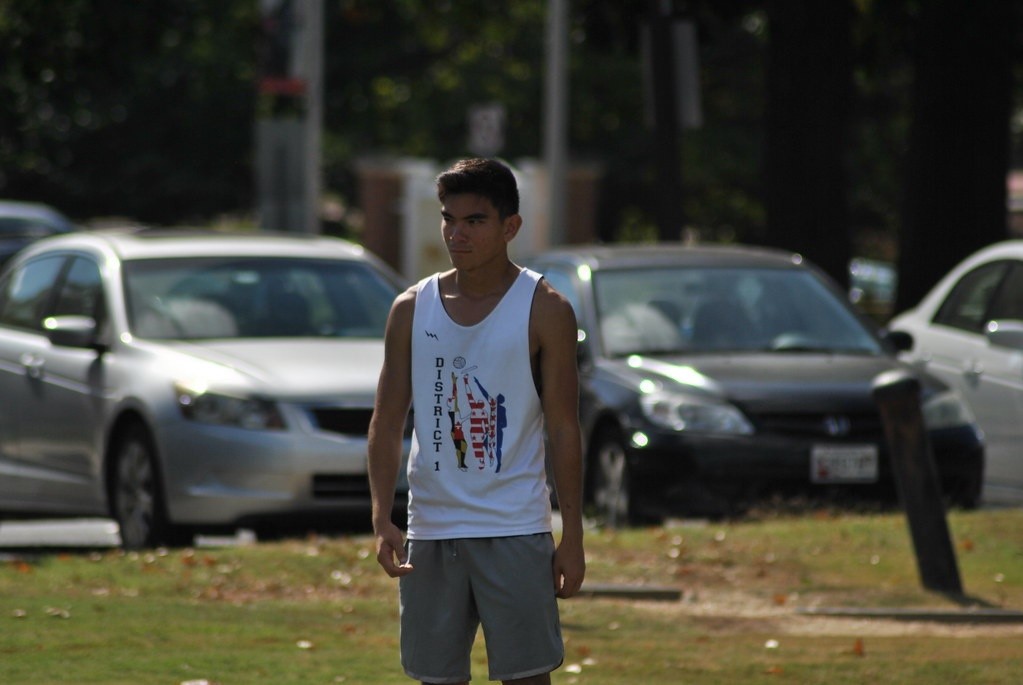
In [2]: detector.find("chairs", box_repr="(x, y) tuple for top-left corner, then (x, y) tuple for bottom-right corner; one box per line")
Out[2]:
(696, 301), (758, 347)
(224, 281), (311, 338)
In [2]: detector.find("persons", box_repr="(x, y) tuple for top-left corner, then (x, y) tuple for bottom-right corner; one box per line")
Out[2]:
(368, 158), (586, 685)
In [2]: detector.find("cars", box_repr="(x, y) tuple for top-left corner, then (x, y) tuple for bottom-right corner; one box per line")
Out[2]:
(529, 242), (987, 531)
(0, 200), (411, 548)
(878, 239), (1023, 506)
